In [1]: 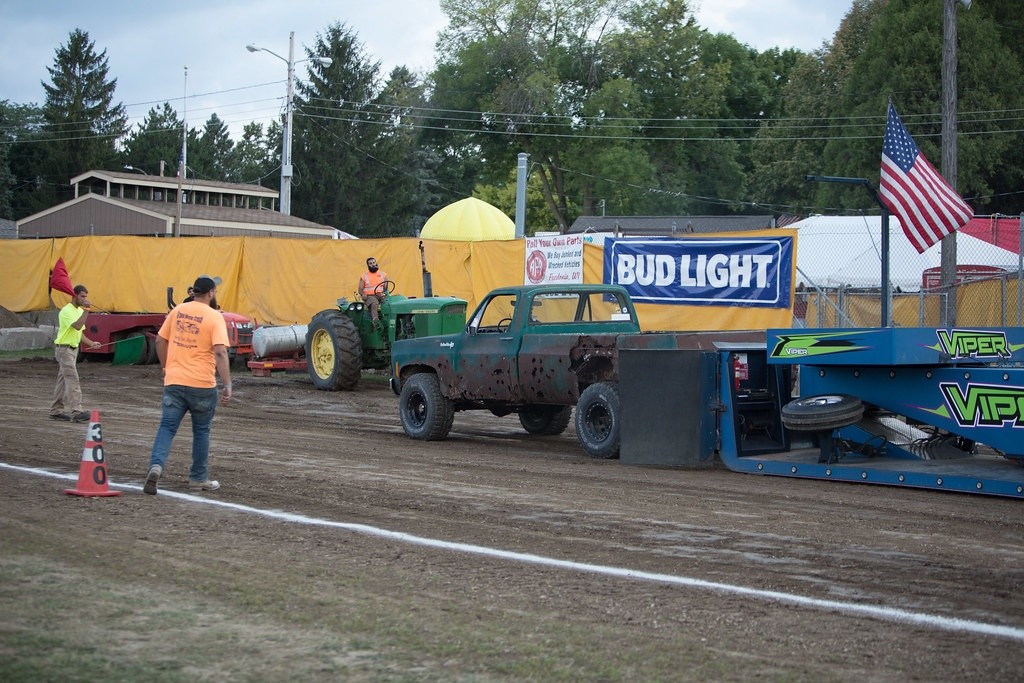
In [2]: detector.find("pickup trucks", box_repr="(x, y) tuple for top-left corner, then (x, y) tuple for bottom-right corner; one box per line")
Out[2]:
(388, 281), (799, 459)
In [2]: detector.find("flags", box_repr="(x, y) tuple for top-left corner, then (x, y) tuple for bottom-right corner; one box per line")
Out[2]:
(880, 102), (976, 253)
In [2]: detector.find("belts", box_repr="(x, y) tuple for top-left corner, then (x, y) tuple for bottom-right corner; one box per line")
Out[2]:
(56, 344), (76, 349)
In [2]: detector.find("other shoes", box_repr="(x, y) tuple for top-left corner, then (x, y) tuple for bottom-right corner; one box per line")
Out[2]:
(143, 467), (161, 495)
(190, 479), (220, 491)
(49, 414), (70, 420)
(372, 317), (381, 332)
(71, 412), (90, 422)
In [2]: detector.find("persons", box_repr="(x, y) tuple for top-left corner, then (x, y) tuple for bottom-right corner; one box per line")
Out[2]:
(143, 277), (232, 495)
(358, 258), (389, 331)
(48, 285), (103, 422)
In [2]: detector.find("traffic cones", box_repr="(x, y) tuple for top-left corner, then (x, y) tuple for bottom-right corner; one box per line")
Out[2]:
(61, 409), (123, 498)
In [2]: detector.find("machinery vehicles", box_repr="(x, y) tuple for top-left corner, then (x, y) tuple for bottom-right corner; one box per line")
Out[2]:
(304, 281), (470, 392)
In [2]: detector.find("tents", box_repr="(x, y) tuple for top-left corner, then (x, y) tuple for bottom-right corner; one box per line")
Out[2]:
(775, 216), (1024, 291)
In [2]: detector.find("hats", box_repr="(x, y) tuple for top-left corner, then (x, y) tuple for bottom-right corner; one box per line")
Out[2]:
(192, 275), (222, 294)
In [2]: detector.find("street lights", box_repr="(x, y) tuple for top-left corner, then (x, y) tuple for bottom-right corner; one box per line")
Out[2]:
(246, 31), (332, 216)
